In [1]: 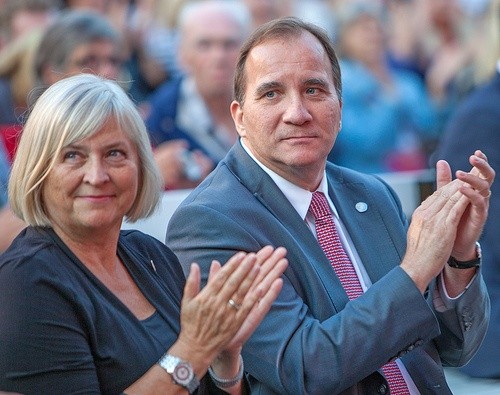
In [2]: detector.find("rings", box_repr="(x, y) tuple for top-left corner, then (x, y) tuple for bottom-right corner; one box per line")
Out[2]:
(484, 189), (492, 201)
(228, 299), (241, 312)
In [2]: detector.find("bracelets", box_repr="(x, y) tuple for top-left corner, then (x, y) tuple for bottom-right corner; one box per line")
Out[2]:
(207, 354), (245, 389)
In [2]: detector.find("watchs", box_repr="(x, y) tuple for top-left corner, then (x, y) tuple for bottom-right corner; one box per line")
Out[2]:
(446, 240), (483, 269)
(158, 353), (201, 395)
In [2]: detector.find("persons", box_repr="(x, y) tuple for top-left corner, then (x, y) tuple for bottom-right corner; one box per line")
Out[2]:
(165, 17), (496, 395)
(0, 72), (288, 395)
(0, 1), (500, 192)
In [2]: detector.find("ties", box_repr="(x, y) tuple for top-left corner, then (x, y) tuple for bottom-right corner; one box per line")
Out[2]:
(309, 191), (412, 394)
